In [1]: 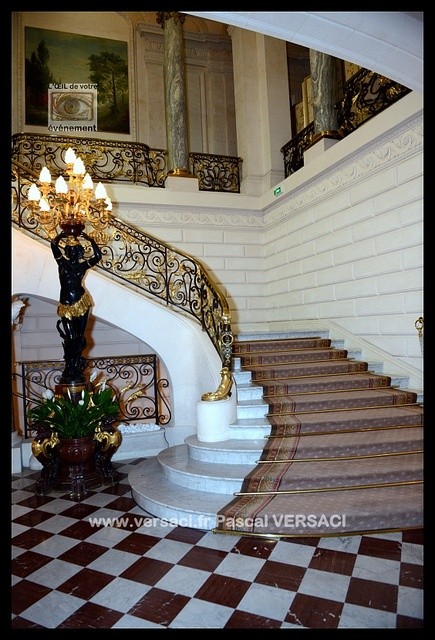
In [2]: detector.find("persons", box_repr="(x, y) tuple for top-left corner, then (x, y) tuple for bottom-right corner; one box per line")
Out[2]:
(51, 231), (102, 384)
(51, 92), (92, 121)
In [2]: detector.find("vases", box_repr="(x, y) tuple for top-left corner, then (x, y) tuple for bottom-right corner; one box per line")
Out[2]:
(35, 437), (118, 498)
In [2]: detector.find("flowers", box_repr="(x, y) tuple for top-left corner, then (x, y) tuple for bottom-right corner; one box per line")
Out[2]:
(25, 371), (120, 442)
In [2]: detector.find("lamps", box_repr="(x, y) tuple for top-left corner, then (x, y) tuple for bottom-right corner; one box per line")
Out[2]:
(24, 142), (116, 394)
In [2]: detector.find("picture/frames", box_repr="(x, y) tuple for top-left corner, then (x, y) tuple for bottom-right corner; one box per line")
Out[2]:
(12, 8), (139, 142)
